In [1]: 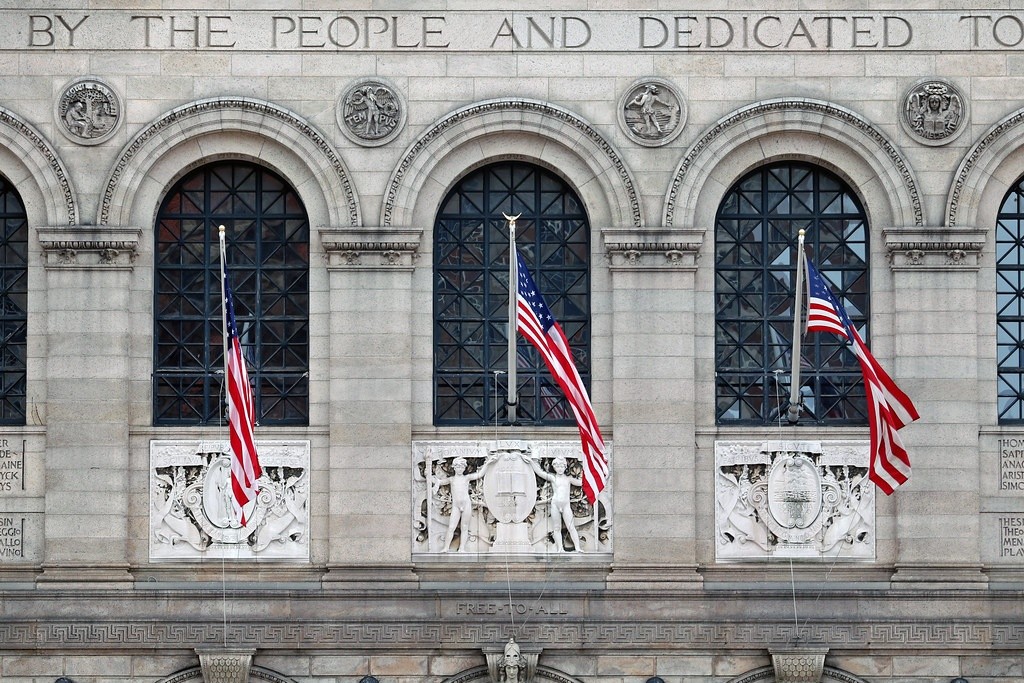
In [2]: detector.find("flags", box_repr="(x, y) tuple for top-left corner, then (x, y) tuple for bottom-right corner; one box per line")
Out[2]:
(515, 251), (610, 505)
(804, 252), (920, 496)
(225, 250), (262, 528)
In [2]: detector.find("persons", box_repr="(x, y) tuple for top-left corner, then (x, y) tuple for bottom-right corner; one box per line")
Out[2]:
(525, 455), (582, 554)
(500, 642), (524, 683)
(425, 454), (493, 553)
